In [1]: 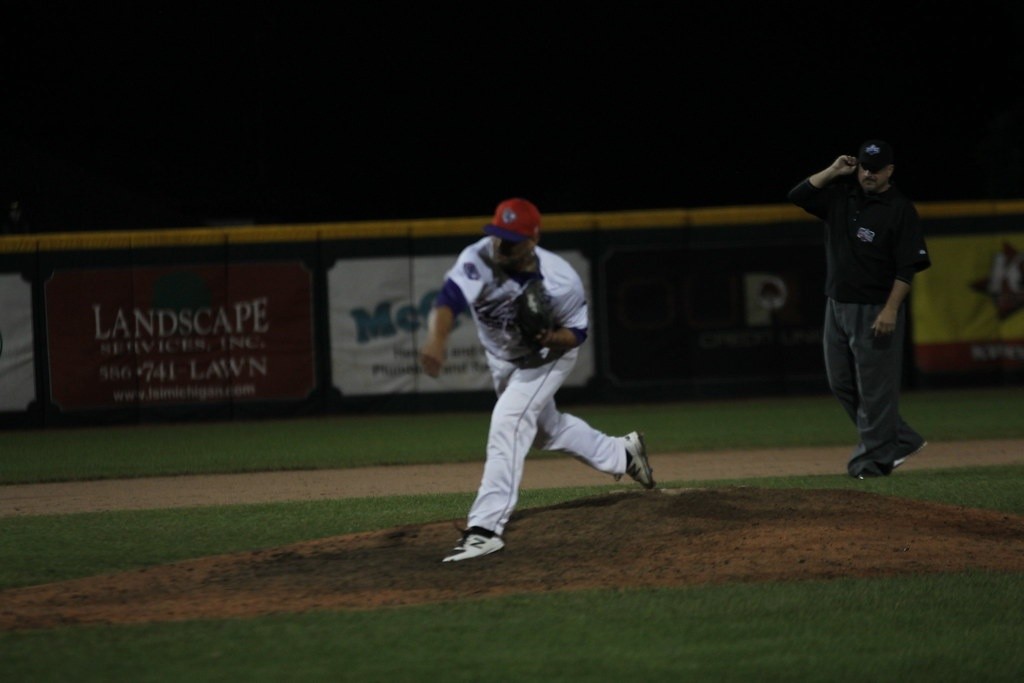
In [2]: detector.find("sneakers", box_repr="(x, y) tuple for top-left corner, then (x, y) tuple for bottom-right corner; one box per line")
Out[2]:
(441, 520), (505, 562)
(615, 431), (655, 489)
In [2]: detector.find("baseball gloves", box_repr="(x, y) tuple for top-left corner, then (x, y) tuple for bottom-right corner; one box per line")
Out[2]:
(512, 279), (555, 343)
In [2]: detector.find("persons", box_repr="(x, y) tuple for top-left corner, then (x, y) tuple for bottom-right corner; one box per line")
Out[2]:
(785, 137), (929, 480)
(418, 199), (657, 563)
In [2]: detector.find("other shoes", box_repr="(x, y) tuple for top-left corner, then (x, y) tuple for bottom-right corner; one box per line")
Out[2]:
(893, 440), (927, 467)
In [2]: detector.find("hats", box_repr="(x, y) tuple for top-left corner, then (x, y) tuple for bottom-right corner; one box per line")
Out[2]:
(484, 198), (540, 240)
(858, 141), (895, 172)
